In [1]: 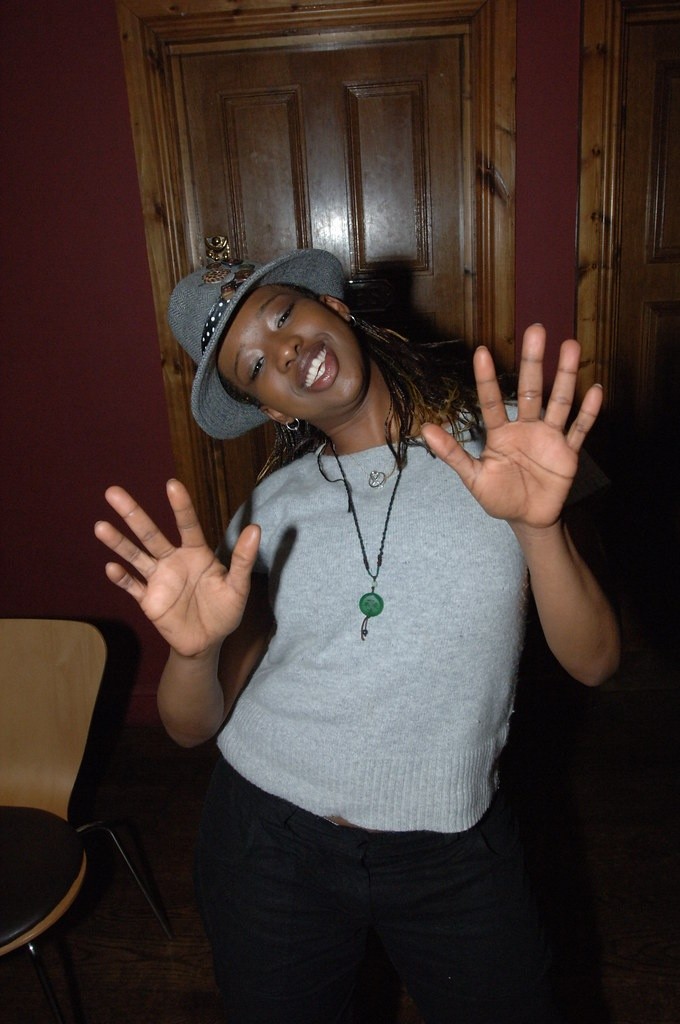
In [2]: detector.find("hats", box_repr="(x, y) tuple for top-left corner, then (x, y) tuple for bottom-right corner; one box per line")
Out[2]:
(167, 235), (346, 441)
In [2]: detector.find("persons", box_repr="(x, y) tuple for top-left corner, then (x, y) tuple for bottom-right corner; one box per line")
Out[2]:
(94, 238), (622, 1024)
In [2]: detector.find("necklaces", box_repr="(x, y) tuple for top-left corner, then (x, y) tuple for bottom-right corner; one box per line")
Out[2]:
(335, 446), (395, 487)
(328, 417), (412, 641)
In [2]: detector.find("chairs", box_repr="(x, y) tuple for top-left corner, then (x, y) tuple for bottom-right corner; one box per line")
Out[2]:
(0, 611), (173, 1024)
